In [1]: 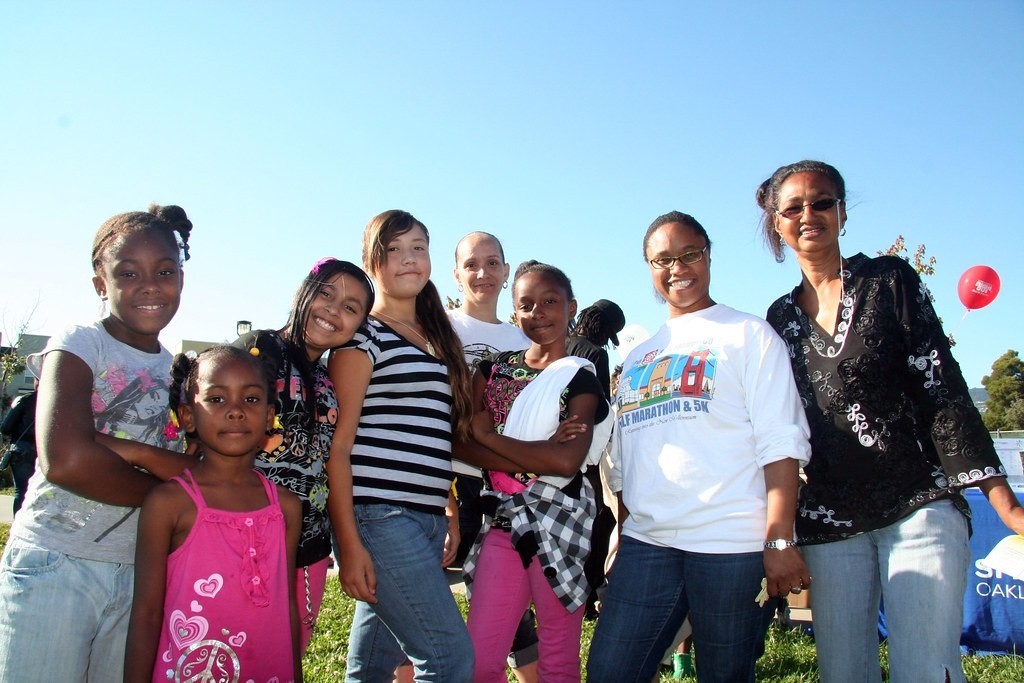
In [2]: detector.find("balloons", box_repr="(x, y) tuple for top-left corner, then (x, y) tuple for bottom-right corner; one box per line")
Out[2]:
(957, 265), (1001, 312)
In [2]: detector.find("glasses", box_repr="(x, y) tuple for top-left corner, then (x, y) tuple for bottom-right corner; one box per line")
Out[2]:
(775, 199), (844, 218)
(647, 243), (708, 268)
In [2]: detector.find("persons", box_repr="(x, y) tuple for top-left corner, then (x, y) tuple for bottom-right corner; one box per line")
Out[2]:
(0, 351), (46, 521)
(395, 231), (550, 683)
(450, 260), (615, 683)
(753, 158), (1024, 683)
(582, 210), (817, 682)
(123, 344), (308, 683)
(327, 208), (477, 683)
(559, 297), (626, 623)
(1, 204), (205, 683)
(230, 256), (377, 662)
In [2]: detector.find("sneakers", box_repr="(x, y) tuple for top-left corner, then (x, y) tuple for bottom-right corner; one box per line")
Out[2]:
(672, 652), (695, 678)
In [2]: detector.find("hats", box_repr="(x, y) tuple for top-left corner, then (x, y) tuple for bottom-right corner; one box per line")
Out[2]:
(592, 299), (625, 346)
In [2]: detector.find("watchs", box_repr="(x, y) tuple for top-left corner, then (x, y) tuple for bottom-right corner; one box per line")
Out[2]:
(763, 538), (796, 551)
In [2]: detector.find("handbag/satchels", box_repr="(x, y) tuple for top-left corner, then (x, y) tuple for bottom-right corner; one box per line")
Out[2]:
(0, 450), (14, 470)
